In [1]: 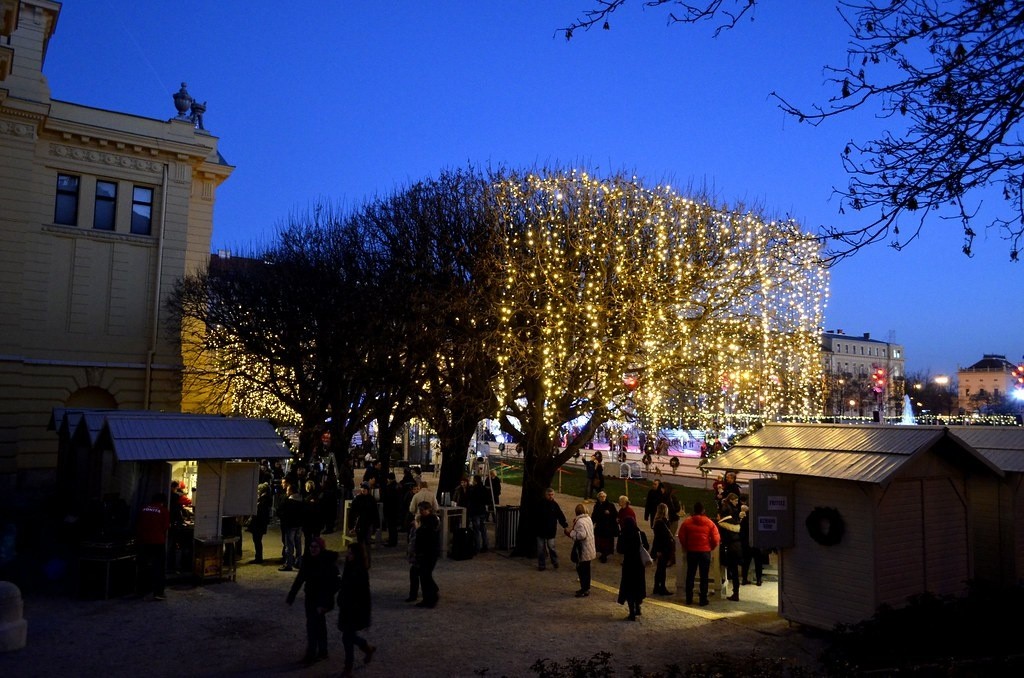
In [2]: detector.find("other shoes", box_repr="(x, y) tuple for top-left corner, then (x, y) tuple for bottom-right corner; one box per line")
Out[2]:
(339, 668), (352, 678)
(686, 599), (694, 605)
(624, 612), (635, 621)
(304, 654), (315, 663)
(278, 567), (292, 571)
(575, 588), (590, 597)
(417, 601), (434, 608)
(700, 601), (710, 606)
(726, 591), (739, 601)
(635, 607), (641, 615)
(742, 579), (751, 585)
(406, 597), (416, 602)
(756, 581), (761, 586)
(659, 590), (674, 595)
(364, 645), (378, 664)
(314, 651), (329, 662)
(652, 590), (658, 594)
(538, 565), (546, 571)
(554, 563), (559, 569)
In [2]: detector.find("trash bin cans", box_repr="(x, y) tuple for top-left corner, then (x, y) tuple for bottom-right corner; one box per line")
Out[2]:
(494, 505), (520, 552)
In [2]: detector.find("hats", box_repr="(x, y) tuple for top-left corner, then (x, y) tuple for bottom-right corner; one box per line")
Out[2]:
(313, 538), (327, 549)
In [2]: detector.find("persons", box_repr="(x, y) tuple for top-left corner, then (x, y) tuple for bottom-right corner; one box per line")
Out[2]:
(261, 422), (514, 572)
(650, 503), (677, 596)
(699, 429), (774, 602)
(409, 513), (440, 609)
(678, 501), (720, 606)
(591, 491), (621, 565)
(555, 421), (682, 567)
(285, 536), (340, 665)
(245, 482), (273, 563)
(530, 487), (571, 570)
(171, 480), (196, 526)
(616, 516), (651, 621)
(404, 501), (441, 605)
(336, 544), (378, 675)
(564, 504), (597, 597)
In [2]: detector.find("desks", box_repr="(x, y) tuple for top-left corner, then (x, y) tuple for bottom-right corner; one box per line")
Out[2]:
(342, 499), (384, 550)
(437, 507), (467, 561)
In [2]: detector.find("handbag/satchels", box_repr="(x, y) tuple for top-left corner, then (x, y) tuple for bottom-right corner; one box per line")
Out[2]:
(571, 532), (583, 563)
(638, 531), (653, 568)
